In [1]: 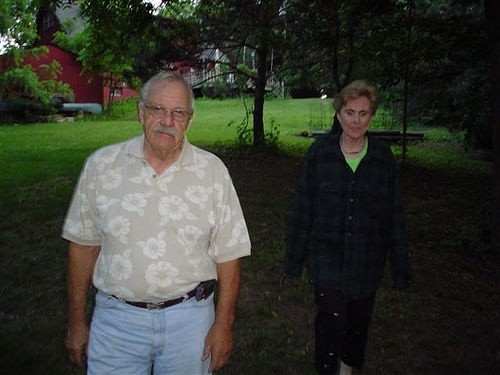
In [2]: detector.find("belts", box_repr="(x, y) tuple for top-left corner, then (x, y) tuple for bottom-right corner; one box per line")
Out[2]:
(111, 289), (199, 310)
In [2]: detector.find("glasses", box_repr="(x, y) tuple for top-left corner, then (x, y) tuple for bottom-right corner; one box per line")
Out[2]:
(144, 102), (194, 122)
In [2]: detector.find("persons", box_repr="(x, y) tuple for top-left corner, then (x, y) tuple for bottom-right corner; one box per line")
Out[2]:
(283, 81), (408, 375)
(60, 71), (251, 375)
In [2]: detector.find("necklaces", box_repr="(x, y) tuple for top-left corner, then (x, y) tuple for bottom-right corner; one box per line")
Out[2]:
(340, 134), (365, 154)
